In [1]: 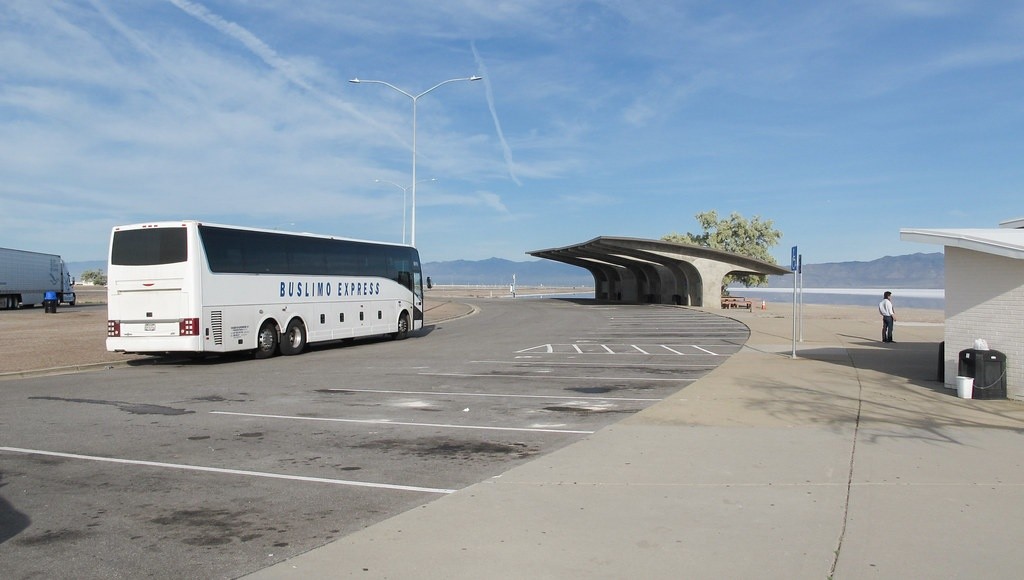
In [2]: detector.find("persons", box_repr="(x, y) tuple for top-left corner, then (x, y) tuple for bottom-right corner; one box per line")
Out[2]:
(879, 291), (897, 344)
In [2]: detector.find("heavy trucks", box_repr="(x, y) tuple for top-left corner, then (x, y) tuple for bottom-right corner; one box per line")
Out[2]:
(0, 247), (77, 309)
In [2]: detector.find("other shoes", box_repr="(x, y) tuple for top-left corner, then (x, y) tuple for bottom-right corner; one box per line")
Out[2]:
(889, 341), (896, 343)
(883, 341), (888, 343)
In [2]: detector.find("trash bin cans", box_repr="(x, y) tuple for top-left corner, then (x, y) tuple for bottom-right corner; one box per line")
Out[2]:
(44, 290), (58, 314)
(958, 348), (1007, 399)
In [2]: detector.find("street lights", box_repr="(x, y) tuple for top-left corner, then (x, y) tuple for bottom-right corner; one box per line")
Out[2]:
(347, 75), (483, 249)
(374, 178), (437, 246)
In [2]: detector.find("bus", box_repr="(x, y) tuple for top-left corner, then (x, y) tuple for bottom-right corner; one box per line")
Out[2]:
(105, 220), (430, 360)
